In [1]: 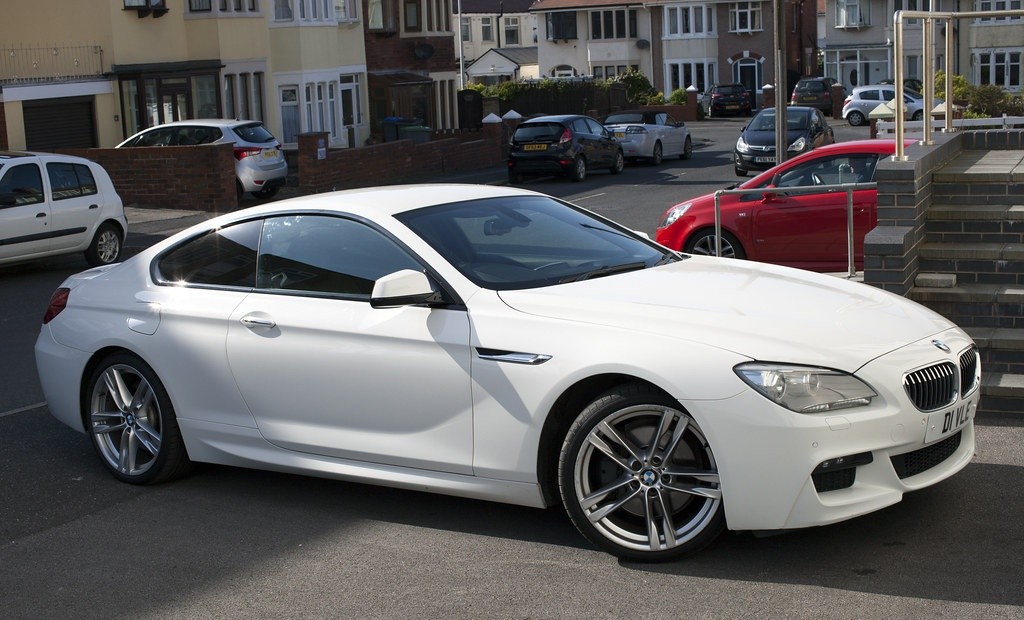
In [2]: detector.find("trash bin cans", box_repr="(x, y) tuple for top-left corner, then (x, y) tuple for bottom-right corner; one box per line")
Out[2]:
(383, 117), (433, 144)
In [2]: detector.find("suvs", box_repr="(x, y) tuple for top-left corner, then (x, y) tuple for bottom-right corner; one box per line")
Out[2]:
(790, 76), (847, 119)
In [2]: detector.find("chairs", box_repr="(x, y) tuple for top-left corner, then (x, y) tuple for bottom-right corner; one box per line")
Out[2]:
(424, 215), (477, 265)
(284, 233), (361, 293)
(855, 154), (879, 183)
(800, 116), (806, 128)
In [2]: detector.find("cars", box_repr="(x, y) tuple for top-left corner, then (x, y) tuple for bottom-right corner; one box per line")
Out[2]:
(652, 138), (921, 273)
(732, 106), (835, 178)
(115, 118), (289, 199)
(504, 114), (625, 182)
(877, 79), (924, 93)
(33, 184), (984, 568)
(841, 85), (946, 127)
(0, 151), (129, 269)
(702, 83), (753, 118)
(603, 111), (693, 166)
